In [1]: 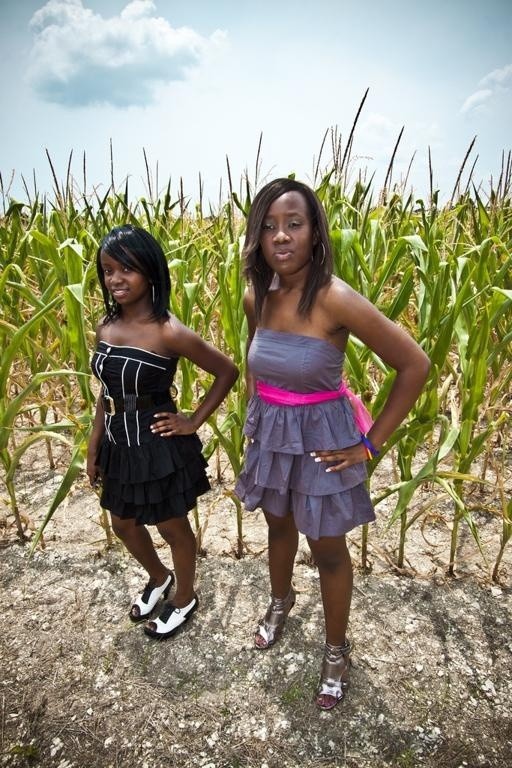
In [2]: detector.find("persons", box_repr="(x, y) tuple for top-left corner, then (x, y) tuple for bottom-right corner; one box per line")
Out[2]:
(83, 223), (242, 643)
(237, 177), (432, 714)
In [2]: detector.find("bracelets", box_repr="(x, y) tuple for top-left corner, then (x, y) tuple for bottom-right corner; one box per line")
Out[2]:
(360, 432), (380, 461)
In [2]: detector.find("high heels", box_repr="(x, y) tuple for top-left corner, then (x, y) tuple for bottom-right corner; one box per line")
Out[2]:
(143, 591), (201, 641)
(127, 566), (175, 623)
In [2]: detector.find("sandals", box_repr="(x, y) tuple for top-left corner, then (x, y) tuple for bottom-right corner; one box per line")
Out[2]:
(253, 587), (296, 653)
(314, 635), (356, 713)
